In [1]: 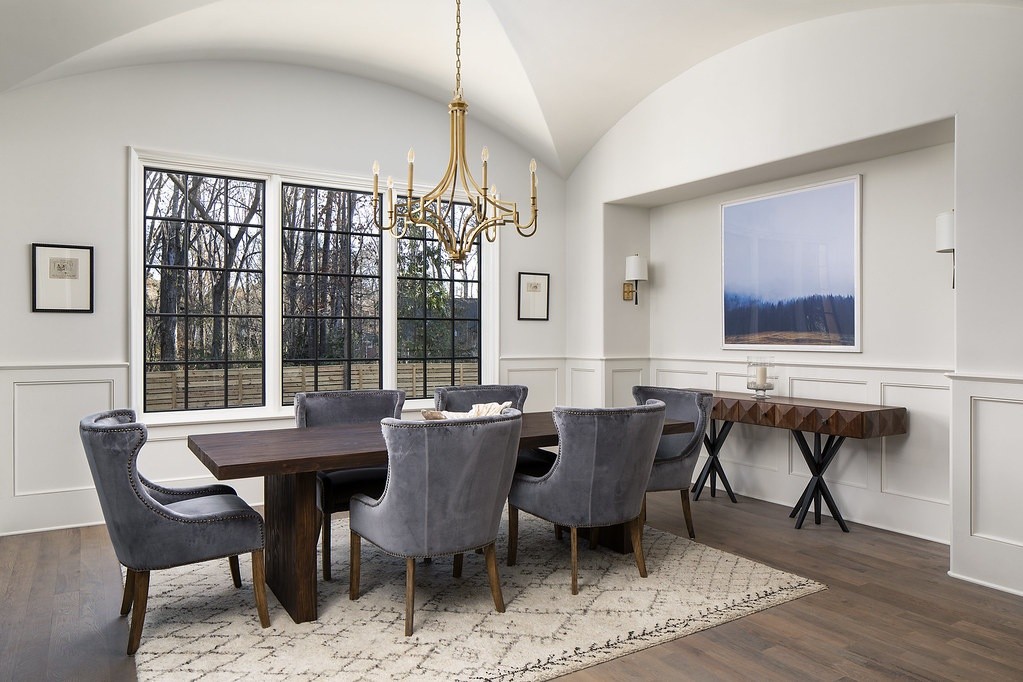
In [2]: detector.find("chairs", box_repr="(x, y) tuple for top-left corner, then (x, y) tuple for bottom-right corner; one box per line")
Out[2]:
(647, 384), (715, 540)
(293, 389), (406, 579)
(347, 408), (524, 635)
(79, 407), (270, 658)
(507, 399), (668, 592)
(434, 384), (529, 413)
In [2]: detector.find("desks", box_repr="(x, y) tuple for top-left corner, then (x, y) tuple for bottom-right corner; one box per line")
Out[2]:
(686, 388), (909, 531)
(187, 411), (697, 626)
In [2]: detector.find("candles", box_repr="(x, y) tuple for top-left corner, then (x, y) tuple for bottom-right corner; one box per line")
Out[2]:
(755, 366), (767, 385)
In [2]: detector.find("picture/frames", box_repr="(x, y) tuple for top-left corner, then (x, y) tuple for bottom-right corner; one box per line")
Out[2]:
(32, 242), (95, 314)
(517, 271), (550, 321)
(721, 173), (864, 354)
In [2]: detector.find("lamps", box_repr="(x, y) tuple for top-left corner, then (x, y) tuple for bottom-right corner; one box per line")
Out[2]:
(936, 209), (956, 290)
(371, 0), (540, 261)
(624, 254), (649, 306)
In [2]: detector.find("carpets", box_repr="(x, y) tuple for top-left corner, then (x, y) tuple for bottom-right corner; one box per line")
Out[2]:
(117, 504), (829, 682)
